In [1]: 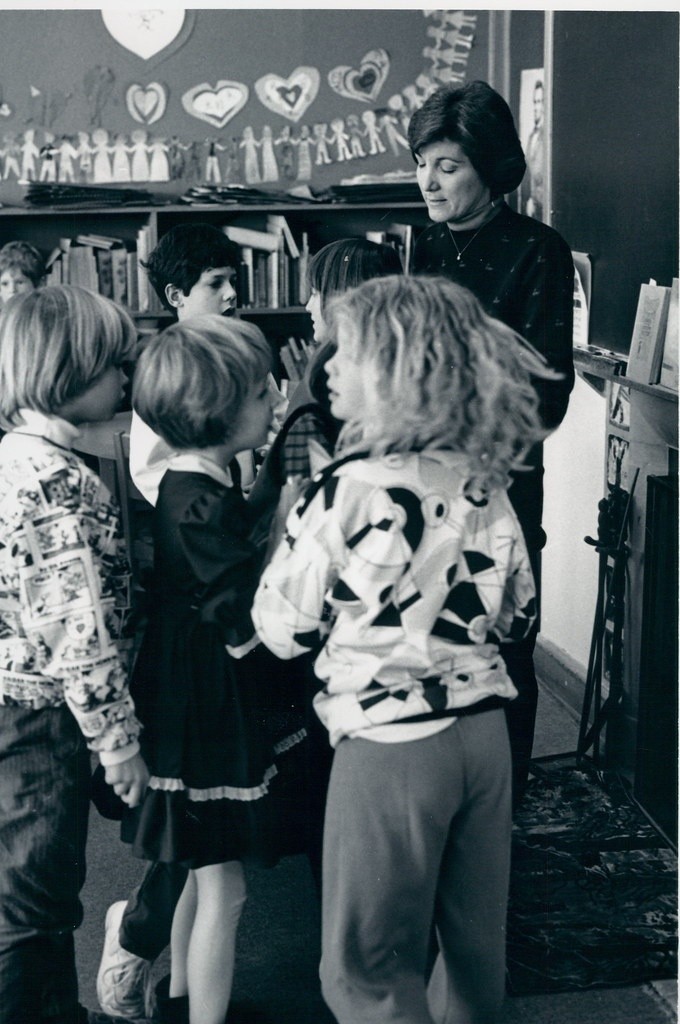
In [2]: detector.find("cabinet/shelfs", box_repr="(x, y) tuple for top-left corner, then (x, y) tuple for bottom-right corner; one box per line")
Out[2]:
(0, 202), (429, 336)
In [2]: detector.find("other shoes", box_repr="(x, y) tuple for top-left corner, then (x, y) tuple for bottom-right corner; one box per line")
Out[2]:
(87, 1009), (129, 1023)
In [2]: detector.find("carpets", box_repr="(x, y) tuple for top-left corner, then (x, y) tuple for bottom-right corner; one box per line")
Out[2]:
(507, 751), (678, 997)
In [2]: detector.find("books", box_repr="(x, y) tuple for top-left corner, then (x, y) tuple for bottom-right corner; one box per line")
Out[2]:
(625, 278), (677, 394)
(42, 215), (412, 400)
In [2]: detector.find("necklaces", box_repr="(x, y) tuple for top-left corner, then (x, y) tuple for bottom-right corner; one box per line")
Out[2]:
(446, 221), (484, 260)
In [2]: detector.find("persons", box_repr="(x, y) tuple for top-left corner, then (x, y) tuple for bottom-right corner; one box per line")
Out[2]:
(96, 312), (308, 1024)
(98, 382), (274, 1024)
(251, 275), (563, 1024)
(0, 239), (42, 302)
(128, 224), (285, 508)
(250, 238), (406, 577)
(0, 285), (150, 1024)
(408, 80), (576, 821)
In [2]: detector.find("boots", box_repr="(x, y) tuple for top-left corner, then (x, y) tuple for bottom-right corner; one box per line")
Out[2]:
(155, 973), (191, 1024)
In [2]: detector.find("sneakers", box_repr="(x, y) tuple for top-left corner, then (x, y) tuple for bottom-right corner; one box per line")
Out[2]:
(96, 902), (152, 1023)
